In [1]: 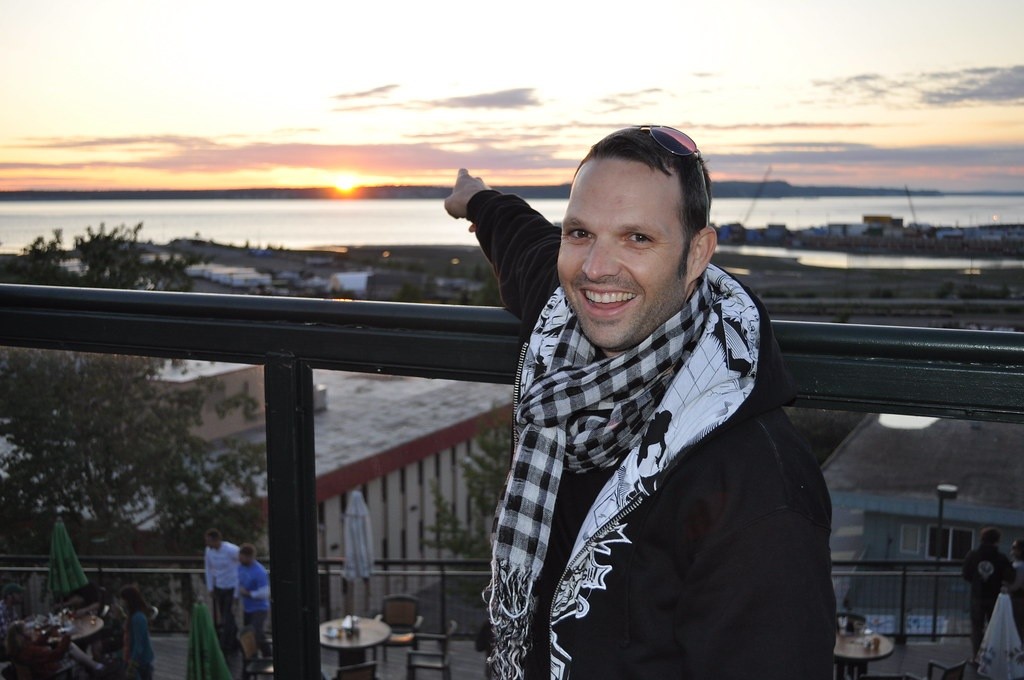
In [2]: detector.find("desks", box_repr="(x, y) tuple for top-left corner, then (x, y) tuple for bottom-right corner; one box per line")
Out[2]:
(319, 616), (392, 669)
(27, 614), (104, 654)
(834, 630), (895, 680)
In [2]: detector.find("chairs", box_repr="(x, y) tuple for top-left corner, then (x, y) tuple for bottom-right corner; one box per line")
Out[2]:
(372, 595), (424, 662)
(406, 619), (457, 680)
(906, 657), (968, 680)
(237, 625), (274, 680)
(330, 662), (382, 680)
(835, 613), (866, 680)
(7, 653), (80, 680)
(109, 604), (159, 658)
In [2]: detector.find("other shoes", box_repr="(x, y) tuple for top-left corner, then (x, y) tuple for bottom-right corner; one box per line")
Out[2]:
(93, 662), (118, 679)
(968, 657), (980, 668)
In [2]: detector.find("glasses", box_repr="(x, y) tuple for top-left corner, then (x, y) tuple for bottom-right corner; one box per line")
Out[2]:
(1009, 544), (1016, 551)
(625, 124), (710, 228)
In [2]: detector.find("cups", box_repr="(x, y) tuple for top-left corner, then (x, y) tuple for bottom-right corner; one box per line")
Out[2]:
(873, 638), (880, 650)
(336, 628), (343, 638)
(89, 611), (98, 625)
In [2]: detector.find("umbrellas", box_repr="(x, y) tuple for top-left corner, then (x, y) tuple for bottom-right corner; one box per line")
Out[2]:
(186, 598), (232, 680)
(976, 586), (1024, 680)
(46, 518), (88, 606)
(340, 490), (371, 615)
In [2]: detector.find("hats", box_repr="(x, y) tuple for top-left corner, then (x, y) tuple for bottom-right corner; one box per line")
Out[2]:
(3, 583), (26, 592)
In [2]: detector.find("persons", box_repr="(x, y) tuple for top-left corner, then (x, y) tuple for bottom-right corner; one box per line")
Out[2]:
(444, 125), (837, 680)
(203, 527), (243, 657)
(0, 573), (155, 680)
(962, 526), (1015, 665)
(232, 543), (272, 659)
(1000, 538), (1024, 646)
